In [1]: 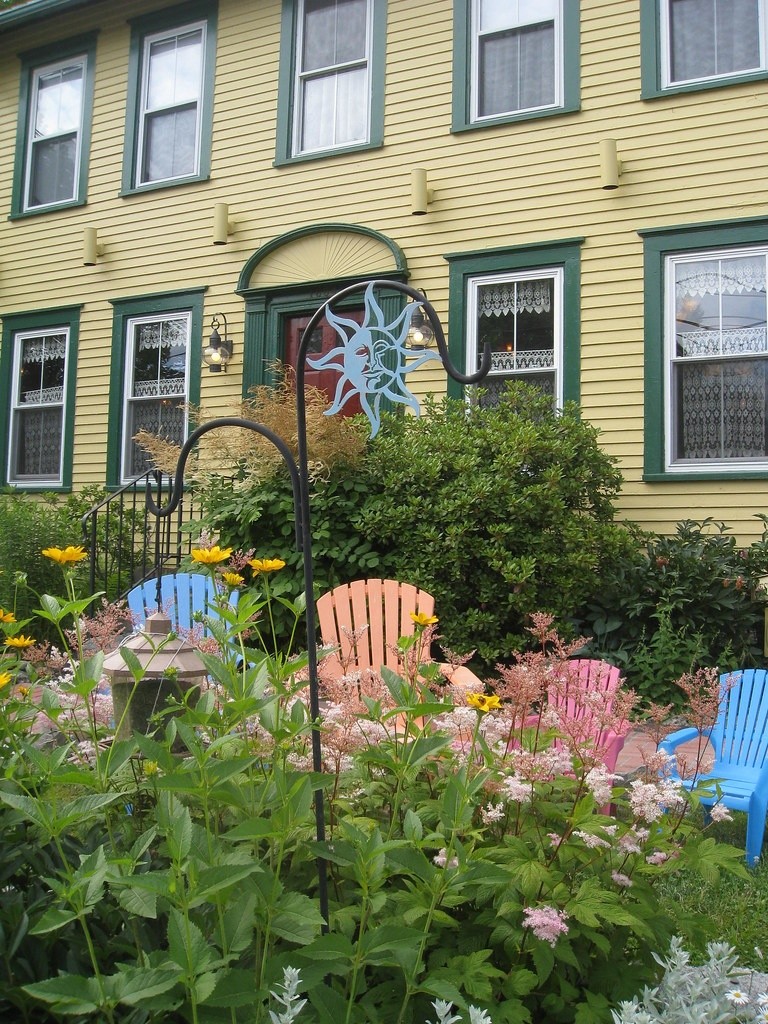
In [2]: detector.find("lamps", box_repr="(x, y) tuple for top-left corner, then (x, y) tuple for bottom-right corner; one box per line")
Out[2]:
(403, 287), (436, 351)
(410, 168), (433, 216)
(598, 137), (622, 191)
(201, 312), (234, 373)
(102, 509), (214, 759)
(82, 227), (107, 265)
(213, 203), (235, 245)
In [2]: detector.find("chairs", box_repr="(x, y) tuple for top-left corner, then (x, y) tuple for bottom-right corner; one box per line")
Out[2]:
(99, 572), (257, 815)
(299, 579), (484, 782)
(507, 659), (632, 816)
(657, 669), (768, 868)
